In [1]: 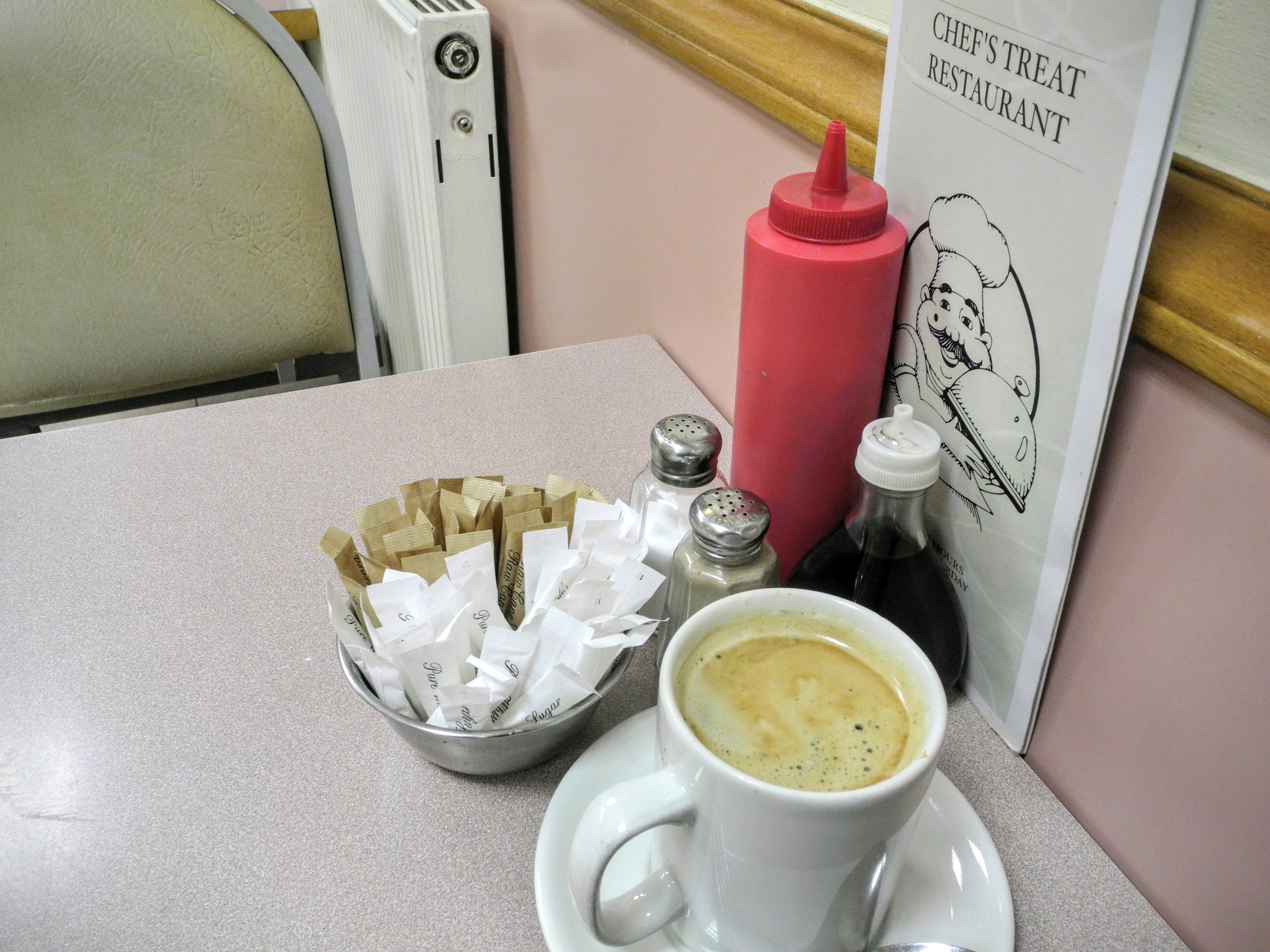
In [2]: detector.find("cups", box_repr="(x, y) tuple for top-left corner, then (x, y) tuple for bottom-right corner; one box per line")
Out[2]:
(570, 586), (947, 952)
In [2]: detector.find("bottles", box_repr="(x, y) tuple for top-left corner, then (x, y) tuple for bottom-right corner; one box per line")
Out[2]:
(783, 403), (968, 693)
(654, 486), (779, 671)
(627, 411), (728, 634)
(730, 121), (908, 582)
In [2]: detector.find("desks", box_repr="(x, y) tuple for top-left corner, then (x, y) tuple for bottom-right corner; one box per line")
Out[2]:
(0, 333), (1193, 951)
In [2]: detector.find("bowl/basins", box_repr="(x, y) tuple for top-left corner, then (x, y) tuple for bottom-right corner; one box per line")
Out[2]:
(336, 597), (636, 776)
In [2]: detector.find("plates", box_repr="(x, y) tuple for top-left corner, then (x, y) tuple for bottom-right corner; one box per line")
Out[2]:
(533, 705), (1014, 952)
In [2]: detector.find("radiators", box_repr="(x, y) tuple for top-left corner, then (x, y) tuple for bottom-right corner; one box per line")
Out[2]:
(314, 1), (510, 374)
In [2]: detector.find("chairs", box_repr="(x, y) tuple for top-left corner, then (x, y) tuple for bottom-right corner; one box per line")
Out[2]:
(0, 1), (382, 436)
